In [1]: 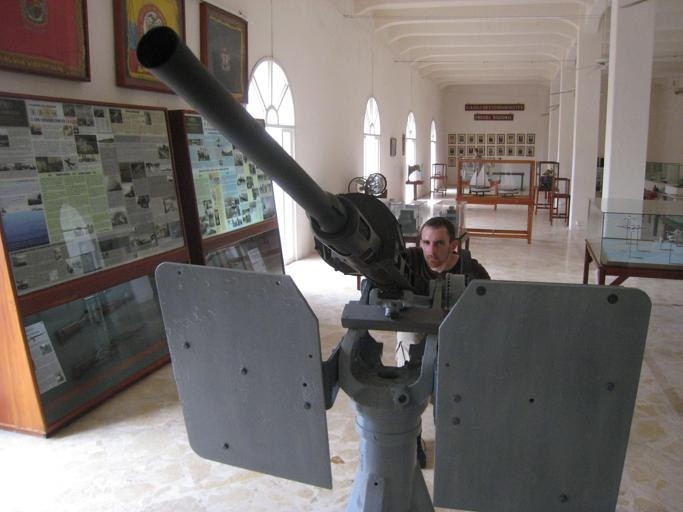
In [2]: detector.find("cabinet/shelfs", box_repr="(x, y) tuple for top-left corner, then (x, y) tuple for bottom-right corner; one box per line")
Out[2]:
(535, 161), (559, 215)
(455, 159), (536, 242)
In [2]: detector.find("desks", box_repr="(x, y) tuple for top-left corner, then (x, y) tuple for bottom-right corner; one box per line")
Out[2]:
(583, 239), (683, 284)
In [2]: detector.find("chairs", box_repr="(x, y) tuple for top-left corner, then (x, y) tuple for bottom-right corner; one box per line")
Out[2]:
(550, 178), (569, 226)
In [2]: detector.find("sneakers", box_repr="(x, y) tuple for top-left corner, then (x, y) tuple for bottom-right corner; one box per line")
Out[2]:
(417, 434), (426, 469)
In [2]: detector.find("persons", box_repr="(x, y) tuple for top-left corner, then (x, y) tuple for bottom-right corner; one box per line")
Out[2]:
(395, 216), (491, 469)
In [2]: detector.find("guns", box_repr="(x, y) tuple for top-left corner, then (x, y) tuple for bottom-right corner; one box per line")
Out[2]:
(134, 26), (651, 512)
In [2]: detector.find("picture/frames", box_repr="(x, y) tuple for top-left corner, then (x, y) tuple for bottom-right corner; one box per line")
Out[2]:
(0, 0), (91, 82)
(114, 0), (189, 95)
(201, 2), (251, 104)
